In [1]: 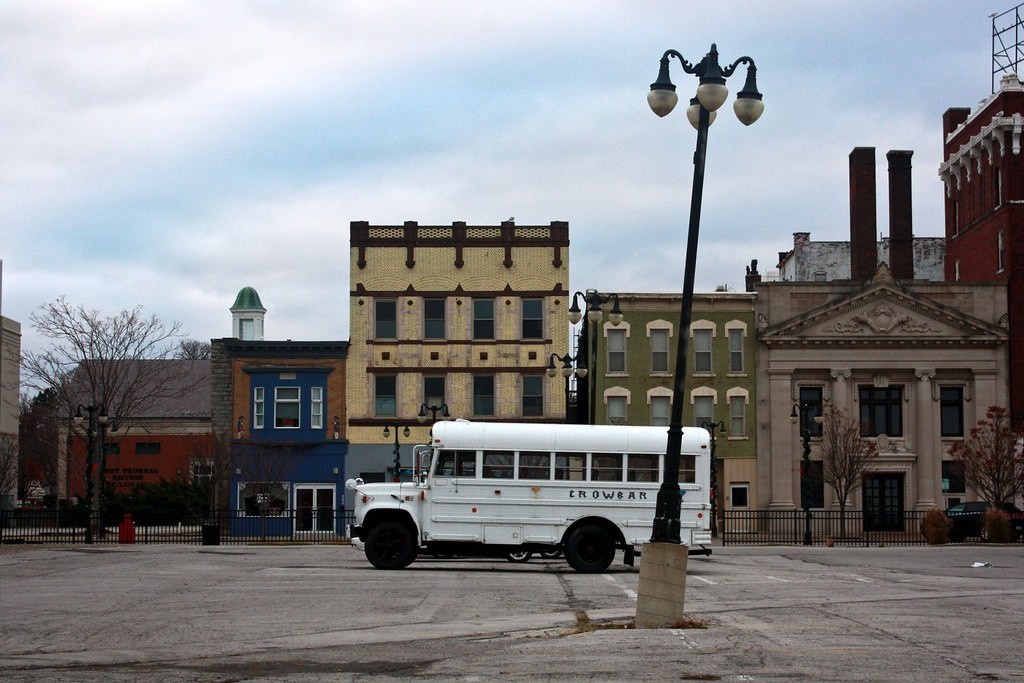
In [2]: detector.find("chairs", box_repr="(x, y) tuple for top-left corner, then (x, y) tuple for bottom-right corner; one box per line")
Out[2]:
(445, 461), (690, 487)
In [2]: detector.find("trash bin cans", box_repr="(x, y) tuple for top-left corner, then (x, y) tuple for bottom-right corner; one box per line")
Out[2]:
(201, 520), (221, 544)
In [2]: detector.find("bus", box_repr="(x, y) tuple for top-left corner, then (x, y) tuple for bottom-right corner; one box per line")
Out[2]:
(346, 418), (712, 574)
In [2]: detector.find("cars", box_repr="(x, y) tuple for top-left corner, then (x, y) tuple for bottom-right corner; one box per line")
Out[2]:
(941, 501), (1023, 542)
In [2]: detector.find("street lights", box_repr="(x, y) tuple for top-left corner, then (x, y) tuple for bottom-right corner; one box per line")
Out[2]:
(566, 289), (624, 425)
(789, 401), (824, 545)
(383, 420), (411, 477)
(546, 352), (588, 425)
(701, 421), (727, 537)
(74, 404), (108, 543)
(644, 42), (765, 545)
(418, 403), (450, 425)
